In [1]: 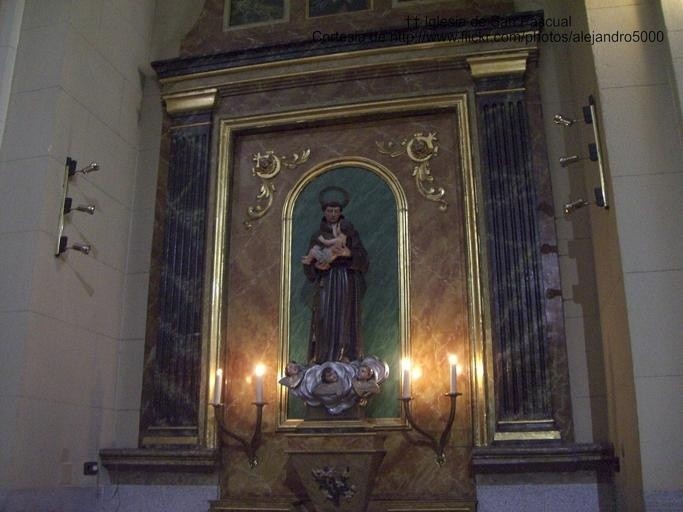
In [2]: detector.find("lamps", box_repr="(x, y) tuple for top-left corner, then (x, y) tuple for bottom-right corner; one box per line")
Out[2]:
(552, 94), (609, 219)
(53, 156), (100, 257)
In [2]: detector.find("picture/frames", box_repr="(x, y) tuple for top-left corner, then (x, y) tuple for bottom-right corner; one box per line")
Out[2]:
(272, 155), (413, 434)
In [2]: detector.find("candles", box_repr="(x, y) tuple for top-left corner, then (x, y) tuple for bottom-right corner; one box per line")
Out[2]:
(254, 368), (263, 402)
(212, 369), (222, 405)
(401, 360), (410, 398)
(448, 356), (457, 394)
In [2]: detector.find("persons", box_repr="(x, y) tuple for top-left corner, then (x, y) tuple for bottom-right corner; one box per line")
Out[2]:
(282, 199), (378, 417)
